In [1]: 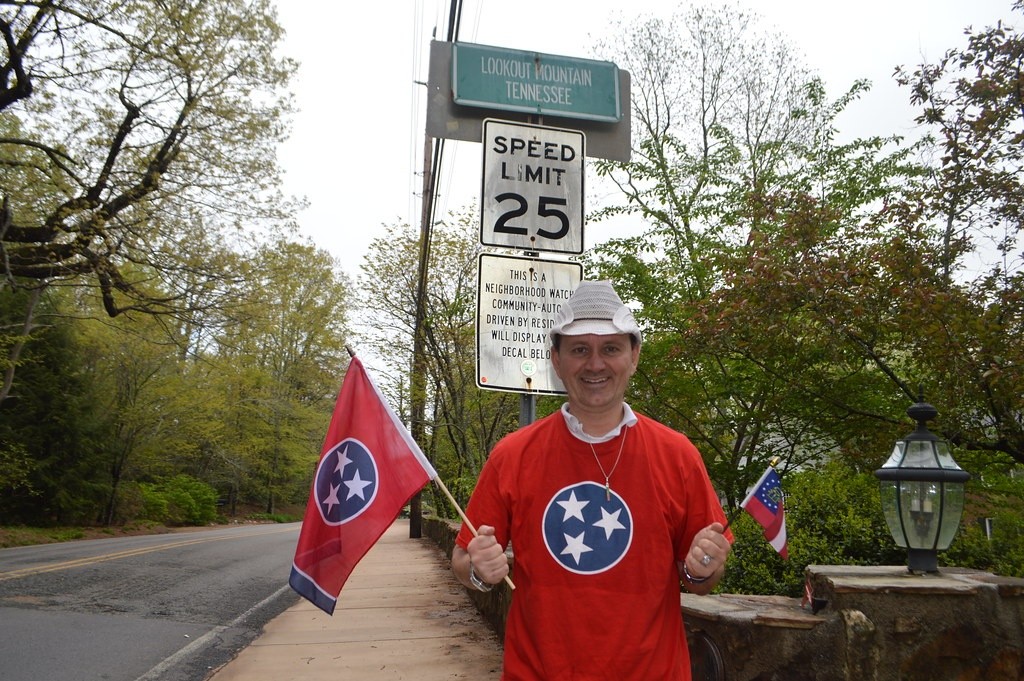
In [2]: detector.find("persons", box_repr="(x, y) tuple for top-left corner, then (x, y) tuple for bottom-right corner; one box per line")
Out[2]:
(452, 280), (735, 681)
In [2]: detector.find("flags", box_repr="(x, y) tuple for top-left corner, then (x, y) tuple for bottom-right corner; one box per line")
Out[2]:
(288, 357), (434, 615)
(742, 468), (787, 559)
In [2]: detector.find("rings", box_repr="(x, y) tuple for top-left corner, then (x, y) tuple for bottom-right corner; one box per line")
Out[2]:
(703, 555), (711, 565)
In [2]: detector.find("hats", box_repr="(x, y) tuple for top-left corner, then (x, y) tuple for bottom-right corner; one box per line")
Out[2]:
(549, 279), (643, 347)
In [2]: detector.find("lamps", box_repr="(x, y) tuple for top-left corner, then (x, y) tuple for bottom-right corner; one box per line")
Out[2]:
(872, 382), (971, 575)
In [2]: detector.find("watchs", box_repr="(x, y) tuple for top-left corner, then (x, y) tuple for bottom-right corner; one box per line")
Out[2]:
(470, 563), (493, 593)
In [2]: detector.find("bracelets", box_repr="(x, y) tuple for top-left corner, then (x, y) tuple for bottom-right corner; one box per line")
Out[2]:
(682, 562), (714, 584)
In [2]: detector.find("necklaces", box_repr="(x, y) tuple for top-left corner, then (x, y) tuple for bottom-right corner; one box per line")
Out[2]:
(588, 424), (627, 503)
